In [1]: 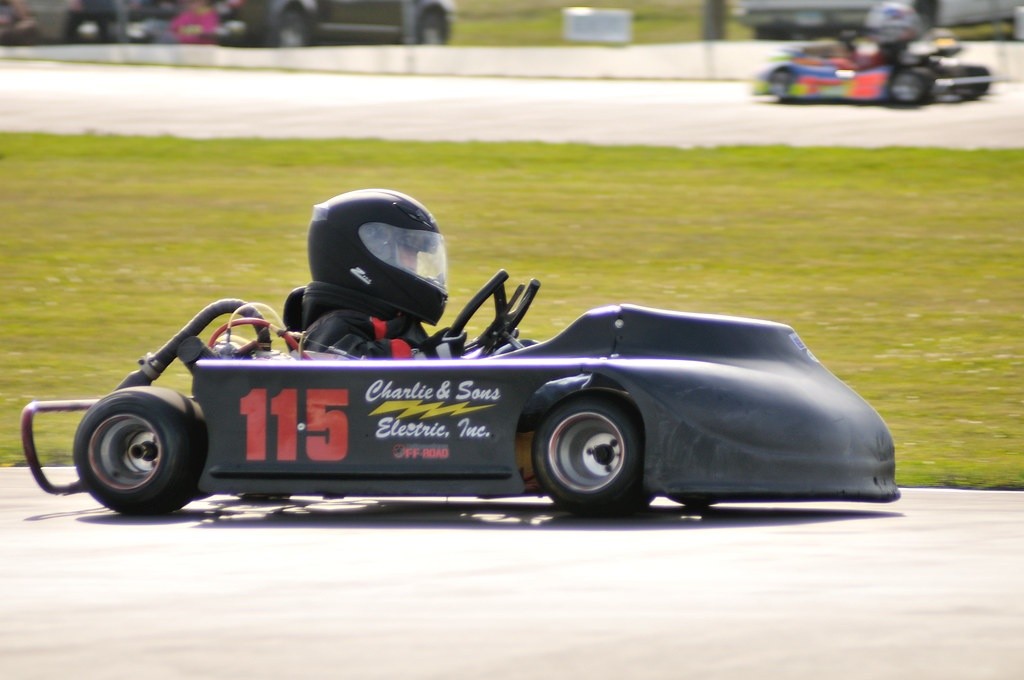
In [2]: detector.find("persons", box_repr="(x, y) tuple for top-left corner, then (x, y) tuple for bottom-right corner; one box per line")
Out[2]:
(835, 1), (926, 70)
(299, 188), (468, 362)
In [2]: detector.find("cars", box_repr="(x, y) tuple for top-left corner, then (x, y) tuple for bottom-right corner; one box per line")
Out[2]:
(734, 0), (1024, 43)
(62, 0), (453, 48)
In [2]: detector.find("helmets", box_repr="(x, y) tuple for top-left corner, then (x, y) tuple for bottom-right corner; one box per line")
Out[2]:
(304, 183), (451, 324)
(864, 0), (926, 44)
